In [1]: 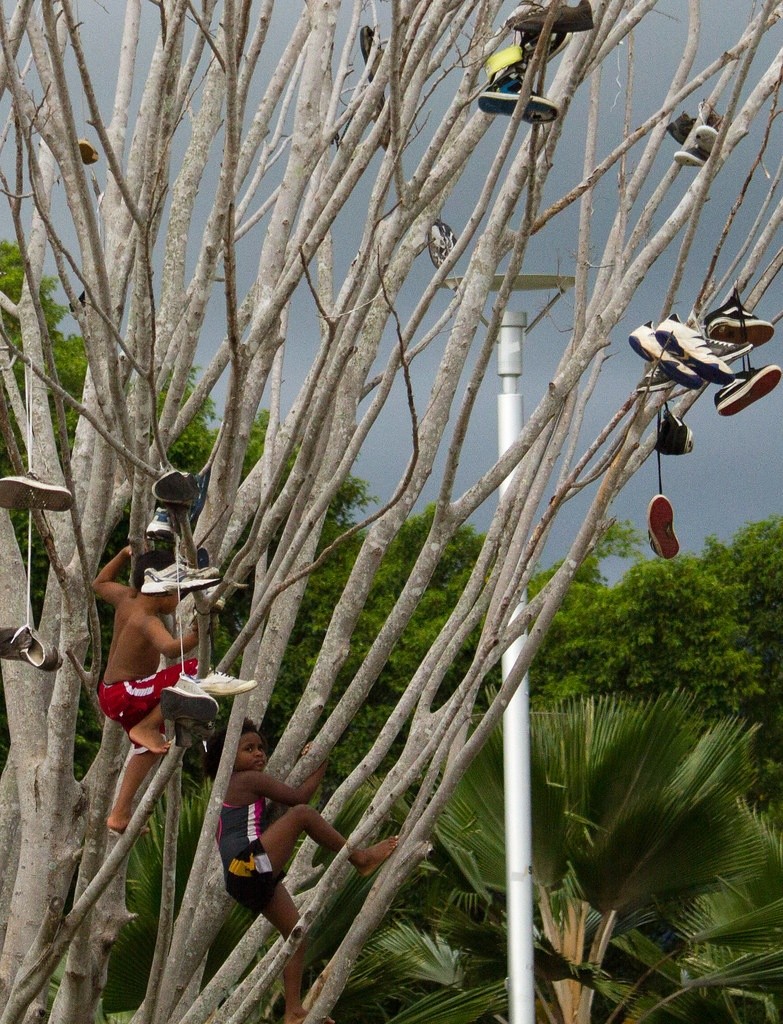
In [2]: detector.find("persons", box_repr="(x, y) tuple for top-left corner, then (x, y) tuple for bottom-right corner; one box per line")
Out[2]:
(202, 717), (398, 1024)
(92, 547), (198, 838)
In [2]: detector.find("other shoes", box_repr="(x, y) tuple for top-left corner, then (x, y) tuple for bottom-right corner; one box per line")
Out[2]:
(78, 139), (99, 165)
(69, 291), (85, 320)
(360, 26), (384, 84)
(175, 718), (215, 748)
(666, 111), (722, 166)
(508, 32), (572, 73)
(428, 220), (457, 269)
(478, 71), (573, 124)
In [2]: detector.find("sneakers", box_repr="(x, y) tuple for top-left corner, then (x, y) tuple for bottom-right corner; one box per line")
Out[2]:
(629, 321), (702, 390)
(0, 626), (62, 671)
(704, 296), (774, 348)
(654, 411), (694, 456)
(201, 584), (226, 609)
(655, 314), (735, 385)
(703, 336), (754, 363)
(141, 562), (220, 597)
(152, 471), (199, 507)
(161, 673), (219, 723)
(648, 494), (679, 560)
(714, 364), (782, 416)
(513, 0), (594, 33)
(146, 506), (175, 544)
(190, 669), (258, 696)
(0, 476), (74, 512)
